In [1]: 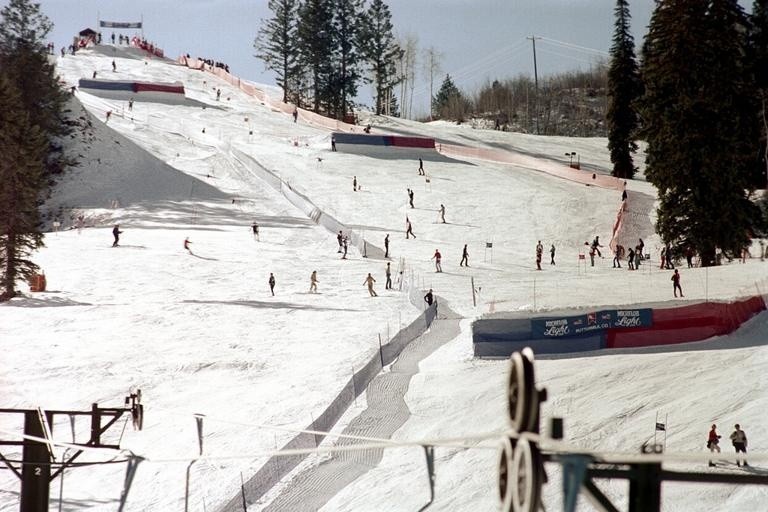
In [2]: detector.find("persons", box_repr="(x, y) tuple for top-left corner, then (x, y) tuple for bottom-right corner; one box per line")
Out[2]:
(331, 136), (337, 151)
(729, 423), (750, 467)
(536, 249), (542, 270)
(293, 108), (298, 122)
(112, 225), (123, 247)
(536, 240), (543, 252)
(46, 31), (230, 122)
(269, 273), (275, 296)
(706, 424), (722, 467)
(586, 235), (693, 270)
(670, 269), (685, 298)
(366, 124), (371, 133)
(251, 222), (260, 241)
(550, 245), (556, 265)
(183, 237), (192, 254)
(337, 158), (470, 305)
(309, 270), (319, 292)
